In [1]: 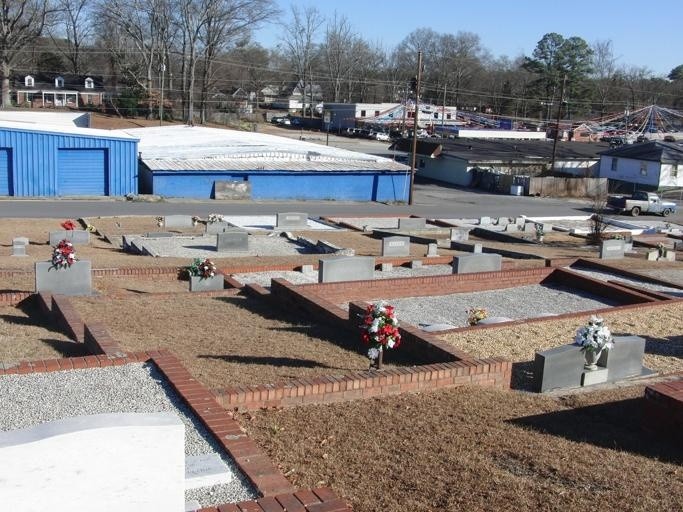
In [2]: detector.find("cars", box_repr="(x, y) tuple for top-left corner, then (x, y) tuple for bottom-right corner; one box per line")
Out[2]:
(270, 116), (292, 127)
(596, 123), (679, 133)
(344, 127), (391, 142)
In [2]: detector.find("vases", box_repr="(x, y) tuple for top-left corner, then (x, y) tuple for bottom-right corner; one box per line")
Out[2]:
(584, 346), (603, 370)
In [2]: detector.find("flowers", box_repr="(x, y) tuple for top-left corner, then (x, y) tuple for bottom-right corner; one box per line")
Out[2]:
(535, 224), (545, 236)
(666, 224), (672, 229)
(573, 314), (617, 355)
(356, 300), (402, 361)
(465, 308), (487, 325)
(49, 239), (80, 270)
(157, 216), (164, 222)
(656, 241), (663, 249)
(208, 212), (224, 224)
(611, 233), (625, 240)
(178, 257), (217, 282)
(62, 218), (77, 232)
(192, 215), (199, 221)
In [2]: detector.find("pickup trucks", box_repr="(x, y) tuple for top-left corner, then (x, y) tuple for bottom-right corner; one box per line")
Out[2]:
(606, 191), (676, 217)
